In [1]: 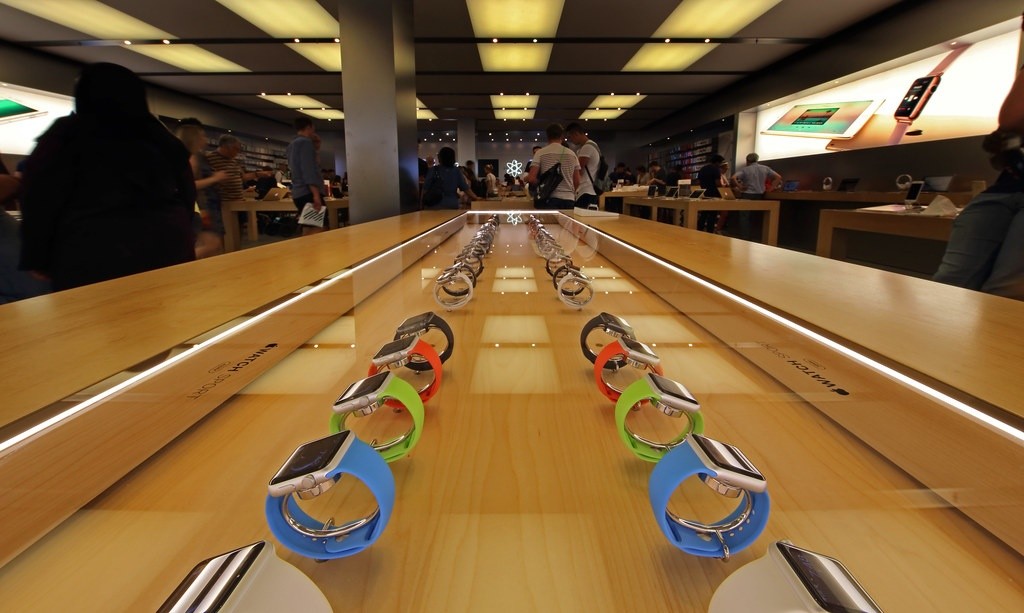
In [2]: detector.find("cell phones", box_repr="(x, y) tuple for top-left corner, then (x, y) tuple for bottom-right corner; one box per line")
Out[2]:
(904, 181), (925, 202)
(0, 97), (48, 125)
(759, 99), (885, 140)
(825, 115), (1001, 151)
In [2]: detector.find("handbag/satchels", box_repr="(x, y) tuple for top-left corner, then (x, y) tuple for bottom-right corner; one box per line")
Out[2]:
(422, 167), (445, 205)
(535, 146), (565, 202)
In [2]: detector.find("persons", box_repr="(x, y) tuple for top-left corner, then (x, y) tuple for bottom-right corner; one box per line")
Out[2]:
(732, 153), (782, 238)
(934, 12), (1024, 300)
(418, 147), (497, 209)
(287, 120), (328, 234)
(698, 155), (739, 233)
(322, 167), (348, 192)
(328, 371), (425, 462)
(524, 123), (602, 209)
(0, 61), (197, 292)
(504, 173), (525, 190)
(177, 118), (277, 259)
(597, 156), (684, 221)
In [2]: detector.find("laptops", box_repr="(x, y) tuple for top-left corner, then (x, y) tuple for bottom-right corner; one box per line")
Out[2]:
(647, 184), (739, 200)
(263, 188), (290, 201)
(781, 180), (801, 192)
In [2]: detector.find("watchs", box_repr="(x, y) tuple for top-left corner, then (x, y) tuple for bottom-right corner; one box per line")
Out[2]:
(649, 433), (770, 557)
(156, 539), (333, 613)
(581, 315), (704, 463)
(265, 431), (396, 560)
(435, 215), (499, 311)
(889, 43), (971, 143)
(529, 215), (593, 308)
(368, 311), (455, 410)
(709, 540), (884, 613)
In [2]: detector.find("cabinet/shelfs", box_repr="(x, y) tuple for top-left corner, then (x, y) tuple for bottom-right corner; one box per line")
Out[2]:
(159, 115), (290, 172)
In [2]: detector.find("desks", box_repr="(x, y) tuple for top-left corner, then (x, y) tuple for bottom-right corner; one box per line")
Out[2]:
(599, 191), (659, 211)
(471, 190), (534, 208)
(224, 198), (349, 253)
(764, 191), (973, 205)
(816, 209), (955, 262)
(623, 196), (780, 246)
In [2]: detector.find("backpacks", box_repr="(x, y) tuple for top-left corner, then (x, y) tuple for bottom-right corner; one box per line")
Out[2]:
(581, 143), (609, 195)
